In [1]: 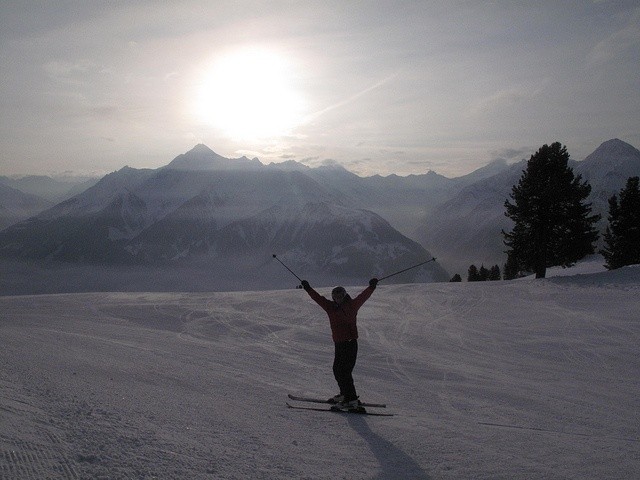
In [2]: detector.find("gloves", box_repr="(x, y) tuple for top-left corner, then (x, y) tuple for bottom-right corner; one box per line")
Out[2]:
(302, 280), (310, 291)
(369, 278), (378, 289)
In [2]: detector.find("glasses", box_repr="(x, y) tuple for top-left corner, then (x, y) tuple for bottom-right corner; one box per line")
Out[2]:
(332, 292), (346, 299)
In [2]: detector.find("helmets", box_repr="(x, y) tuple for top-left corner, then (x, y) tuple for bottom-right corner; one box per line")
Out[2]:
(332, 287), (346, 300)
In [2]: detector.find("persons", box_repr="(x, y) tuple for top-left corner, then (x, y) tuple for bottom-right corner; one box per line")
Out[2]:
(301, 277), (378, 411)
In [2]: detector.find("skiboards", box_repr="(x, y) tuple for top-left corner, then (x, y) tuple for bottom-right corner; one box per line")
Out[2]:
(286, 393), (394, 416)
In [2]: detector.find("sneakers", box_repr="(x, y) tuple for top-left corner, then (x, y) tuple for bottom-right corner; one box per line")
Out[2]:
(336, 398), (358, 412)
(334, 395), (345, 404)
